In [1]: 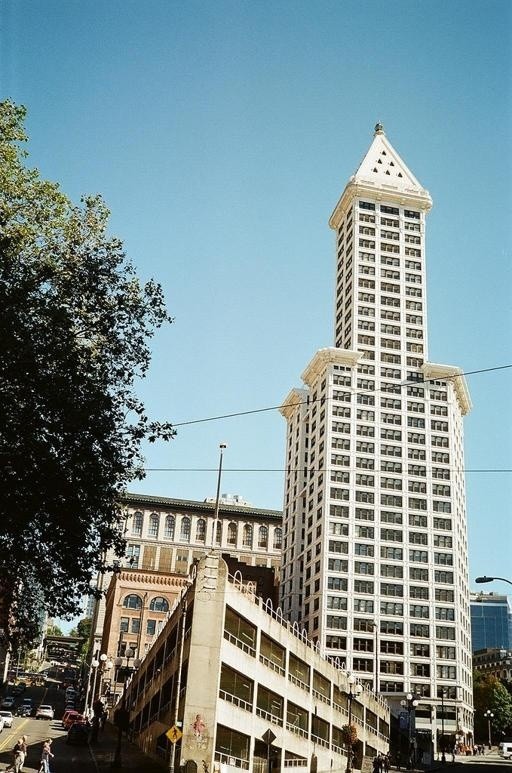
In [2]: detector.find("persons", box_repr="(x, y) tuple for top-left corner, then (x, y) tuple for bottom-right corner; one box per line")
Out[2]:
(386, 751), (392, 769)
(38, 741), (55, 773)
(394, 750), (402, 771)
(457, 742), (486, 755)
(383, 756), (390, 772)
(6, 745), (18, 772)
(373, 757), (379, 772)
(21, 735), (28, 756)
(376, 754), (384, 772)
(13, 738), (26, 772)
(44, 738), (56, 773)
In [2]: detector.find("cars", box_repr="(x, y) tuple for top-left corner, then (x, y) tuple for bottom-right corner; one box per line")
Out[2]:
(0, 661), (90, 747)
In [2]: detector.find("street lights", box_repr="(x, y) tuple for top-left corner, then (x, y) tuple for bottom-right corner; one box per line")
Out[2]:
(484, 710), (496, 750)
(473, 575), (512, 584)
(111, 647), (142, 773)
(91, 653), (113, 746)
(400, 691), (419, 771)
(439, 687), (450, 765)
(336, 672), (364, 773)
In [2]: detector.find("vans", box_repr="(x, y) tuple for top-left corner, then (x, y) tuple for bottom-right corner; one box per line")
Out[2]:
(497, 742), (512, 760)
